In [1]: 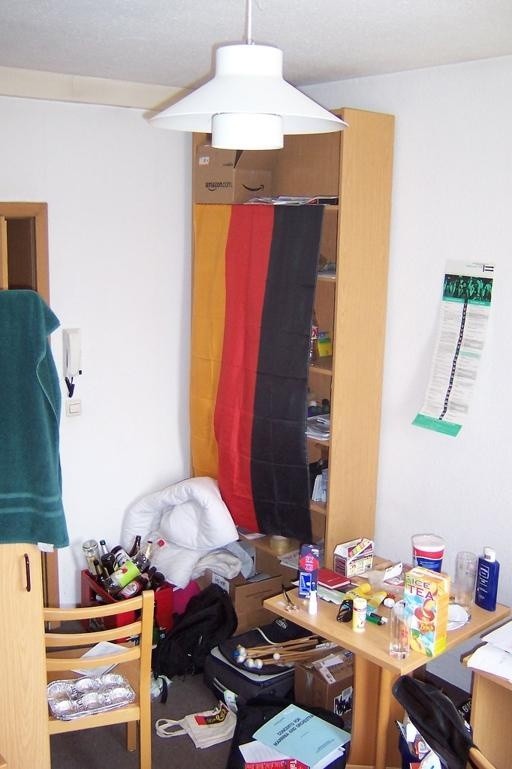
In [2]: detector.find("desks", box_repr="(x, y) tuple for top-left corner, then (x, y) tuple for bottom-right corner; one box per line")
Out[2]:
(261, 553), (511, 769)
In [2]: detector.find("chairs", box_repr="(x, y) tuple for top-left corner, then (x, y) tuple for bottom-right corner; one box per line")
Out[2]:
(42, 589), (154, 769)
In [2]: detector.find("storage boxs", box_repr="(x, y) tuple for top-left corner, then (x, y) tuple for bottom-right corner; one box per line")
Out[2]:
(292, 646), (355, 723)
(203, 568), (283, 636)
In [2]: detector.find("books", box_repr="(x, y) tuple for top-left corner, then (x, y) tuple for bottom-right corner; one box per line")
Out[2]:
(306, 412), (330, 442)
(237, 702), (352, 769)
(290, 566), (358, 605)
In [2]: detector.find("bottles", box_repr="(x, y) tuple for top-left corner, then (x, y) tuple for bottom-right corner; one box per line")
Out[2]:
(306, 396), (329, 418)
(82, 534), (168, 647)
(352, 597), (367, 632)
(308, 316), (319, 363)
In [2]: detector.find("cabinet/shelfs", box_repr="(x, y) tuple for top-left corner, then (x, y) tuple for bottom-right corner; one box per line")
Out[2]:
(186, 105), (397, 567)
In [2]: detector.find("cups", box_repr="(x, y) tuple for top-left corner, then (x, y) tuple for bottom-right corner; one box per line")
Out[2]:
(388, 605), (412, 659)
(453, 552), (478, 609)
(412, 533), (445, 575)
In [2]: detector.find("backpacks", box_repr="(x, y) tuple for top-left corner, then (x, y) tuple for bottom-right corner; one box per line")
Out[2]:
(201, 616), (316, 706)
(151, 581), (239, 681)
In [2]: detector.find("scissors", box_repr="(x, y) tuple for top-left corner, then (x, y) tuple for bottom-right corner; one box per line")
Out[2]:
(283, 584), (299, 612)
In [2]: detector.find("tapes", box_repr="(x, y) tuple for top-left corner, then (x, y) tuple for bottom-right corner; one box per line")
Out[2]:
(269, 535), (292, 552)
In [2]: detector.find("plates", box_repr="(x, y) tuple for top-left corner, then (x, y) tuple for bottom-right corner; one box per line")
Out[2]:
(394, 595), (468, 631)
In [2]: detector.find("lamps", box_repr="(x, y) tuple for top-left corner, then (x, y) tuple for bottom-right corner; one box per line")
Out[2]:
(146, 0), (351, 151)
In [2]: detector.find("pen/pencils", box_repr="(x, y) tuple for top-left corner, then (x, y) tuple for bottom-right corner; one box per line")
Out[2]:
(365, 612), (388, 625)
